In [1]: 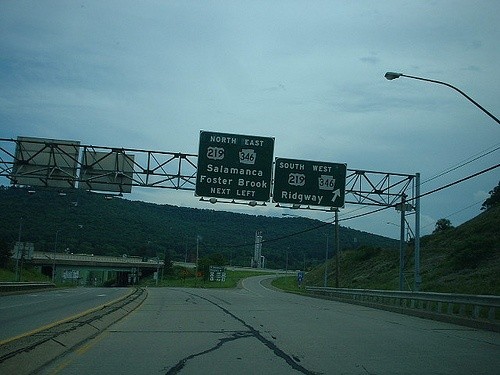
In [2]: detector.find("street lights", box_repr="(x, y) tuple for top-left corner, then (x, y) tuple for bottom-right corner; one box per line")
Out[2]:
(52, 224), (83, 285)
(282, 213), (328, 288)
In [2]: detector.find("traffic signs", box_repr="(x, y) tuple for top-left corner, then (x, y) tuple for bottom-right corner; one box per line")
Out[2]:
(195, 131), (275, 203)
(273, 158), (347, 207)
